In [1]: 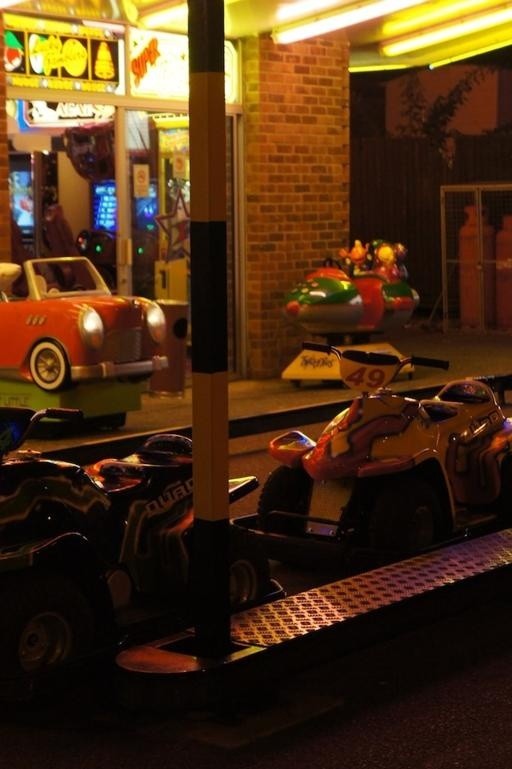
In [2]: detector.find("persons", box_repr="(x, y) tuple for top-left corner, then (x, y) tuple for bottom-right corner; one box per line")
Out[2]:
(8, 176), (15, 208)
